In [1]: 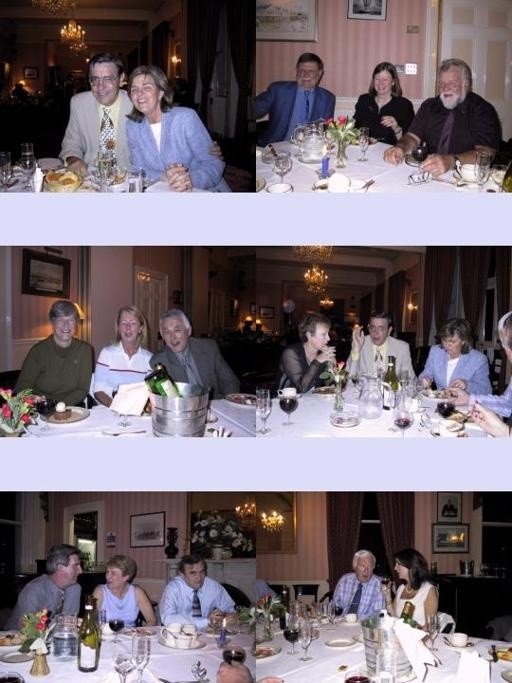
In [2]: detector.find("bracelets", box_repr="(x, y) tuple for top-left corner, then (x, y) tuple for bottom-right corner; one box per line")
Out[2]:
(393, 128), (403, 133)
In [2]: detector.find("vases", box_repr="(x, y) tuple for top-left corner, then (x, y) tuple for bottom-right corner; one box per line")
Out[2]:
(161, 523), (182, 557)
(333, 138), (350, 169)
(29, 646), (53, 678)
(332, 381), (348, 412)
(260, 613), (278, 643)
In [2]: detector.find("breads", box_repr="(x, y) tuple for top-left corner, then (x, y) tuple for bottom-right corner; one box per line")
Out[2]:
(46, 171), (78, 186)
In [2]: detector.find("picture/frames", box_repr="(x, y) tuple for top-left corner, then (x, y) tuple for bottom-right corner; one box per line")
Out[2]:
(434, 491), (466, 523)
(125, 508), (167, 549)
(19, 246), (73, 300)
(256, 0), (323, 45)
(249, 301), (256, 315)
(258, 305), (276, 319)
(346, 0), (390, 22)
(22, 65), (40, 80)
(426, 522), (470, 555)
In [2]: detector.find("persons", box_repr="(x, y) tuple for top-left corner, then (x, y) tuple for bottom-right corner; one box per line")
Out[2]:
(442, 499), (456, 516)
(59, 48), (223, 192)
(381, 547), (438, 631)
(93, 555), (157, 626)
(353, 61), (415, 144)
(326, 550), (389, 617)
(383, 56), (501, 180)
(346, 312), (415, 380)
(470, 399), (512, 437)
(5, 543), (84, 631)
(94, 304), (157, 406)
(216, 659), (253, 683)
(14, 300), (92, 407)
(125, 63), (234, 192)
(417, 317), (492, 396)
(256, 52), (336, 148)
(257, 676), (285, 683)
(445, 310), (512, 417)
(272, 312), (336, 398)
(255, 578), (281, 601)
(150, 308), (240, 400)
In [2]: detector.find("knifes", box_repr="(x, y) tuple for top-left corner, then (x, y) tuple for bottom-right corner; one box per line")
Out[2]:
(491, 644), (498, 663)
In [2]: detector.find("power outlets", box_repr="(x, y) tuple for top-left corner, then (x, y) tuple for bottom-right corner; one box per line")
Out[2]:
(404, 60), (418, 75)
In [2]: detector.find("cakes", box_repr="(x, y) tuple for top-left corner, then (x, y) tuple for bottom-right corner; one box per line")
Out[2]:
(54, 402), (72, 420)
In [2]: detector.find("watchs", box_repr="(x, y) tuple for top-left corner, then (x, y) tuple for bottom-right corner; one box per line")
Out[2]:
(454, 155), (460, 168)
(159, 555), (238, 631)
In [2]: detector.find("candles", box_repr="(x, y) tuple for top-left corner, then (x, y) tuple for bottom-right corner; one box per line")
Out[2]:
(220, 616), (229, 631)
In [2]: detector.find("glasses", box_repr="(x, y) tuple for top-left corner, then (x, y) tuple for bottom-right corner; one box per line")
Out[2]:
(88, 71), (120, 87)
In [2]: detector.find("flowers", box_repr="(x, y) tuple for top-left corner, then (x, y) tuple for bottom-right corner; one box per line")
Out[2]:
(320, 114), (363, 147)
(0, 384), (37, 437)
(16, 603), (58, 651)
(317, 358), (361, 386)
(256, 592), (285, 620)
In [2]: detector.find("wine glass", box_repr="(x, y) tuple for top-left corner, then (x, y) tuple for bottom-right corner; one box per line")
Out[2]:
(38, 395), (53, 433)
(113, 634), (151, 683)
(392, 403), (412, 437)
(308, 598), (338, 633)
(278, 393), (299, 426)
(271, 153), (293, 183)
(116, 396), (133, 429)
(400, 365), (430, 411)
(412, 145), (433, 173)
(472, 153), (491, 193)
(0, 139), (143, 192)
(255, 389), (270, 436)
(281, 622), (314, 662)
(108, 614), (127, 645)
(355, 127), (370, 165)
(379, 574), (390, 599)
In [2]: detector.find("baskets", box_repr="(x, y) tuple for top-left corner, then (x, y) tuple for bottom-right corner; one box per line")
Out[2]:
(41, 166), (83, 192)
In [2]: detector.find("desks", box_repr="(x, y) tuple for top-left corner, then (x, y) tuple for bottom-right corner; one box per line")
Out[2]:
(256, 385), (511, 439)
(256, 127), (512, 191)
(254, 619), (511, 683)
(24, 392), (255, 439)
(1, 155), (218, 192)
(1, 616), (256, 682)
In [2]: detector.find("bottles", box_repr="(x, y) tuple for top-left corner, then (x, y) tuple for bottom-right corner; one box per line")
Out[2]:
(143, 363), (178, 398)
(49, 614), (76, 664)
(27, 646), (52, 678)
(77, 596), (100, 671)
(400, 600), (414, 621)
(383, 354), (398, 411)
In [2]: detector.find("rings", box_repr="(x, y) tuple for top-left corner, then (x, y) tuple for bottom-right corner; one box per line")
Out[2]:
(173, 172), (179, 177)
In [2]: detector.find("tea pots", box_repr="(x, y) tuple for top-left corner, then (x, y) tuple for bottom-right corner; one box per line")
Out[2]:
(289, 120), (323, 163)
(355, 374), (393, 420)
(161, 621), (183, 646)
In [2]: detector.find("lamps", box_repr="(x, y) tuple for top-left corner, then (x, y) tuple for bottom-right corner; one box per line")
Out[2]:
(171, 55), (182, 64)
(291, 246), (339, 310)
(259, 496), (292, 534)
(58, 15), (95, 63)
(232, 491), (256, 530)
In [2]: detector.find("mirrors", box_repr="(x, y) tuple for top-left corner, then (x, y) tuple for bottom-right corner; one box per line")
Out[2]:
(256, 493), (296, 556)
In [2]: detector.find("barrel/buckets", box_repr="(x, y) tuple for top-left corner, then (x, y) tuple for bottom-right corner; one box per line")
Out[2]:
(362, 615), (419, 683)
(150, 382), (210, 437)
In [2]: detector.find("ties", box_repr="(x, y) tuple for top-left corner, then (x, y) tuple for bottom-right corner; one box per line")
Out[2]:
(436, 110), (456, 156)
(191, 587), (202, 618)
(51, 591), (65, 620)
(304, 90), (311, 126)
(347, 582), (363, 613)
(181, 353), (201, 387)
(375, 348), (383, 380)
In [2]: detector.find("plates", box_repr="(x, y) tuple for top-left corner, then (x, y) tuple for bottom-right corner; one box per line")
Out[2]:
(314, 178), (369, 193)
(500, 669), (512, 683)
(225, 390), (257, 405)
(429, 424), (490, 437)
(40, 403), (90, 424)
(488, 646), (512, 662)
(121, 627), (156, 637)
(0, 649), (31, 663)
(422, 389), (455, 402)
(326, 639), (356, 648)
(442, 639), (473, 649)
(256, 643), (282, 661)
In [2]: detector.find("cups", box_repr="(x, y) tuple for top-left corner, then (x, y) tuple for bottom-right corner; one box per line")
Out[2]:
(374, 646), (397, 683)
(438, 420), (462, 437)
(266, 183), (294, 194)
(222, 644), (247, 666)
(455, 158), (479, 184)
(344, 612), (360, 622)
(438, 394), (454, 418)
(275, 388), (299, 396)
(459, 560), (474, 576)
(451, 630), (469, 645)
(489, 163), (510, 193)
(174, 633), (191, 650)
(345, 669), (372, 683)
